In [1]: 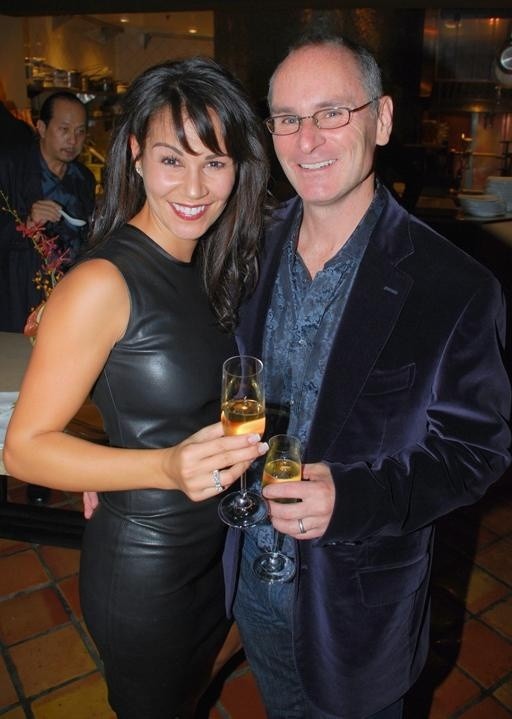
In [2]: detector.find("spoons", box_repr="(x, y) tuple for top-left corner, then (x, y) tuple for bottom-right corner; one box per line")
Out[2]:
(57, 209), (86, 227)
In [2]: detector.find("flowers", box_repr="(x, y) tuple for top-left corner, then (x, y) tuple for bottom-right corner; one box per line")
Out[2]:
(0, 190), (65, 311)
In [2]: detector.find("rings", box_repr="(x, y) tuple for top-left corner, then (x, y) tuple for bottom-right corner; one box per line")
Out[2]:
(297, 519), (305, 533)
(212, 469), (224, 493)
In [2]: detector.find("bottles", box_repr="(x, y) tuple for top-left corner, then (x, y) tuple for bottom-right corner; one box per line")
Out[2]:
(24, 56), (114, 94)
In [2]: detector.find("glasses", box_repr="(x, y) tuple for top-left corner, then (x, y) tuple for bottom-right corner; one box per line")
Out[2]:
(262, 93), (382, 137)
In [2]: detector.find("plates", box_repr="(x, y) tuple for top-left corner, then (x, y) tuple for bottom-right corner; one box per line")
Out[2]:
(455, 173), (512, 218)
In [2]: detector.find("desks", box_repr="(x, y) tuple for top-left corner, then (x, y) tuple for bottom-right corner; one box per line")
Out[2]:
(1, 331), (105, 548)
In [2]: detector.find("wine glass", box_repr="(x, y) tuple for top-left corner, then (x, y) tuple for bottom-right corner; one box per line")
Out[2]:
(219, 356), (304, 585)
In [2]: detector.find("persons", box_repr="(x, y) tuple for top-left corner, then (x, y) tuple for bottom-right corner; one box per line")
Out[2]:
(79, 30), (508, 717)
(0, 90), (98, 337)
(3, 55), (268, 719)
